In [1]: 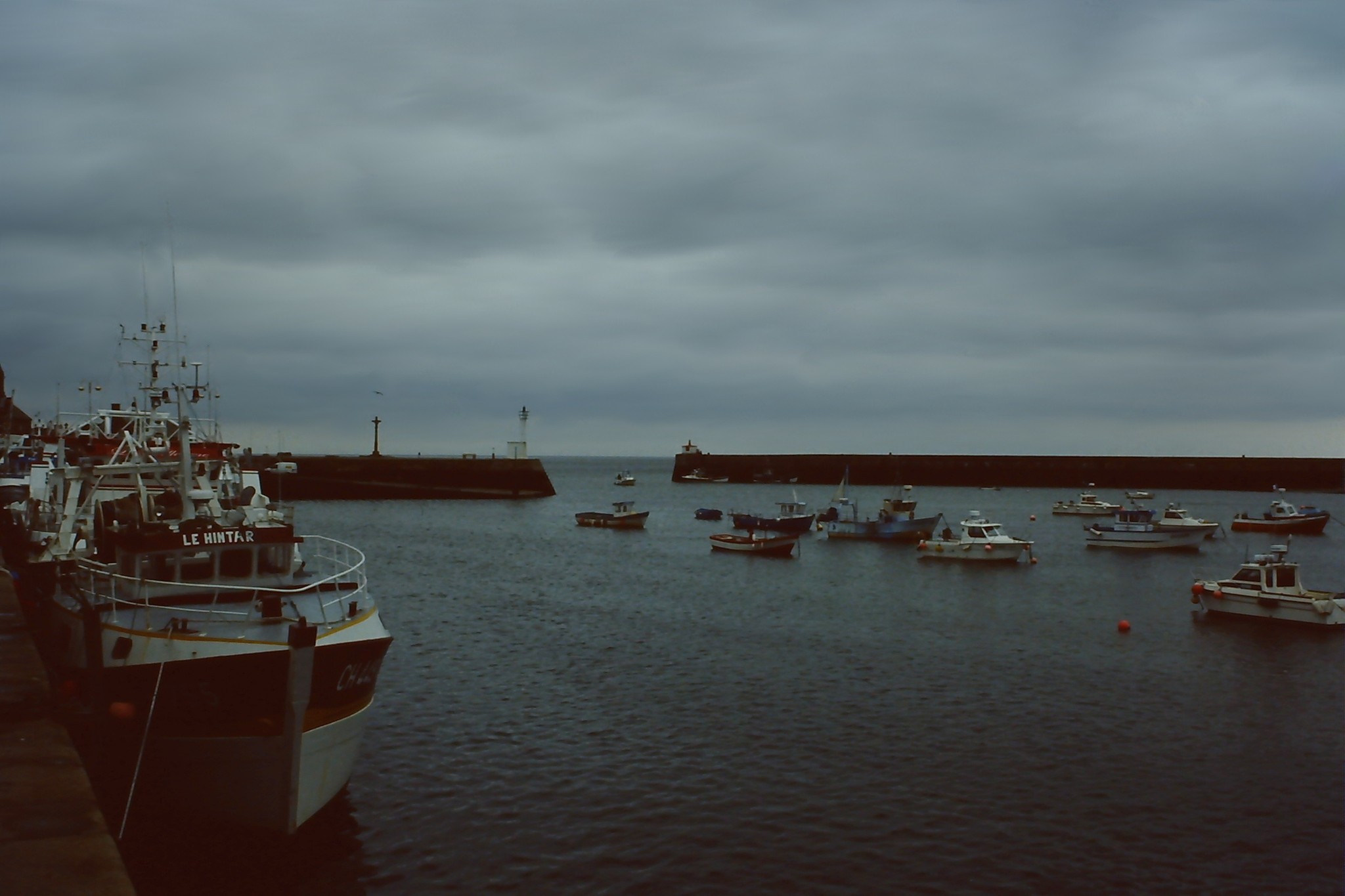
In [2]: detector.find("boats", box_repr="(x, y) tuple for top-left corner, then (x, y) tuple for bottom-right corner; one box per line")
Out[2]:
(0, 261), (394, 842)
(575, 501), (649, 528)
(1083, 489), (1219, 553)
(1052, 483), (1122, 517)
(1195, 533), (1345, 630)
(1125, 490), (1155, 499)
(727, 489), (816, 535)
(709, 526), (801, 557)
(695, 508), (723, 520)
(1231, 485), (1330, 533)
(614, 471), (636, 486)
(819, 465), (942, 544)
(920, 509), (1035, 562)
(681, 468), (729, 482)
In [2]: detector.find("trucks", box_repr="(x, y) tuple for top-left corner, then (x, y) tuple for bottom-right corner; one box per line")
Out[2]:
(265, 462), (298, 475)
